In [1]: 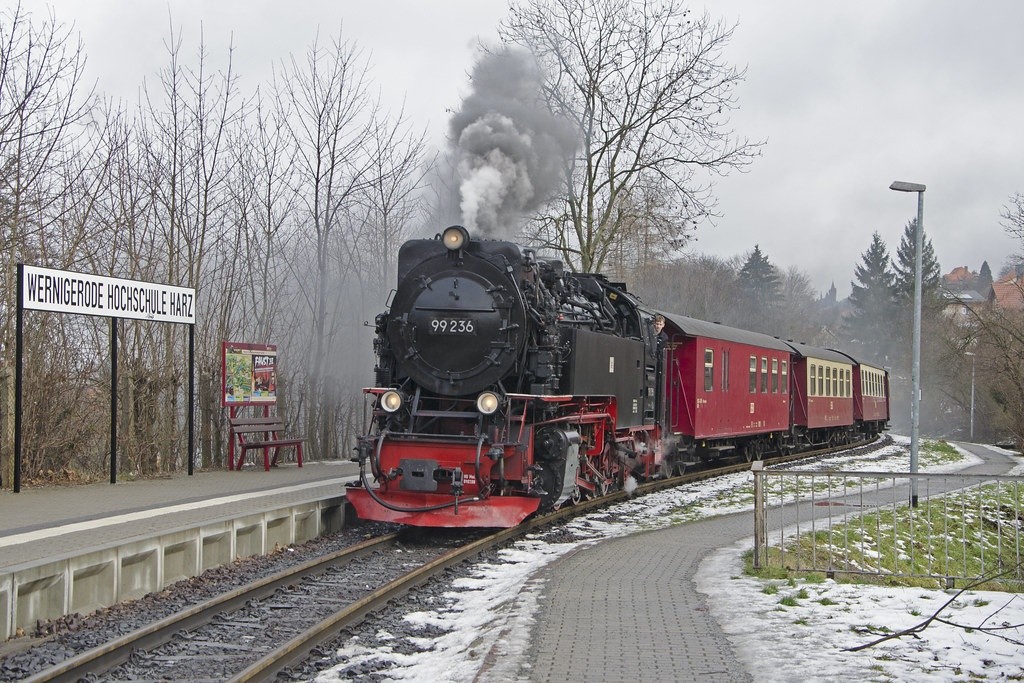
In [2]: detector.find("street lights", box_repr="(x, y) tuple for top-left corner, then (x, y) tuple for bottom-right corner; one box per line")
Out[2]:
(964, 351), (976, 443)
(889, 180), (928, 508)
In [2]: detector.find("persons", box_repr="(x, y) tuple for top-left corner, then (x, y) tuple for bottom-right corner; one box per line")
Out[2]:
(228, 344), (237, 354)
(655, 315), (669, 353)
(226, 384), (234, 396)
(255, 373), (276, 395)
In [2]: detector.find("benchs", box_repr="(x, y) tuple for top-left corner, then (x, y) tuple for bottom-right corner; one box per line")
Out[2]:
(229, 419), (307, 472)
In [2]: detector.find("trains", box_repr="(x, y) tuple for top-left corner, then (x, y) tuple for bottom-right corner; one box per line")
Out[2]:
(341, 223), (892, 529)
